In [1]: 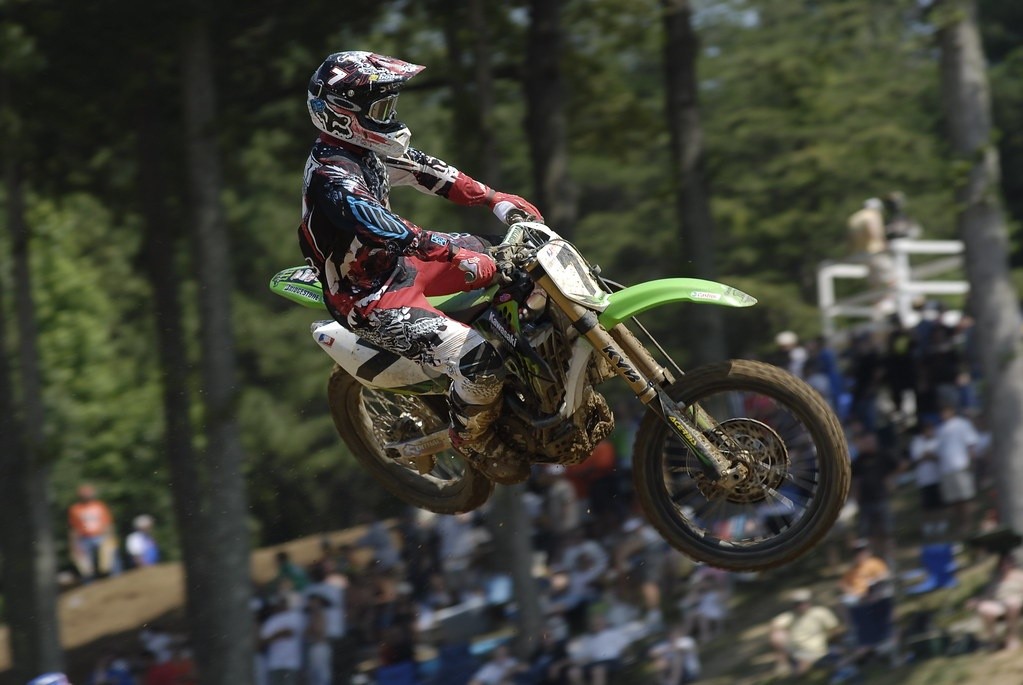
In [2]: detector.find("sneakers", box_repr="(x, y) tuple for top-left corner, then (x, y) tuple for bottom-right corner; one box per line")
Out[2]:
(448, 425), (517, 463)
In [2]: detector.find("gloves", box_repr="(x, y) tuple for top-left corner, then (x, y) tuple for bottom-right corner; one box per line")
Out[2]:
(450, 246), (496, 286)
(488, 191), (545, 228)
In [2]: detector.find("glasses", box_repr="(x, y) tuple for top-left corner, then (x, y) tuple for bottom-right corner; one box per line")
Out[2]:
(368, 89), (400, 124)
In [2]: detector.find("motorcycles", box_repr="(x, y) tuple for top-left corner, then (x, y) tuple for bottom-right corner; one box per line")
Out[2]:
(269, 210), (853, 574)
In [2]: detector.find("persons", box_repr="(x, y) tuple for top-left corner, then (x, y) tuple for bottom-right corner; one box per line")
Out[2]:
(299, 51), (544, 487)
(69, 192), (996, 685)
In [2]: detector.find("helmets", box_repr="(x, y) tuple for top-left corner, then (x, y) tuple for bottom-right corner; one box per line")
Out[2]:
(306, 50), (427, 159)
